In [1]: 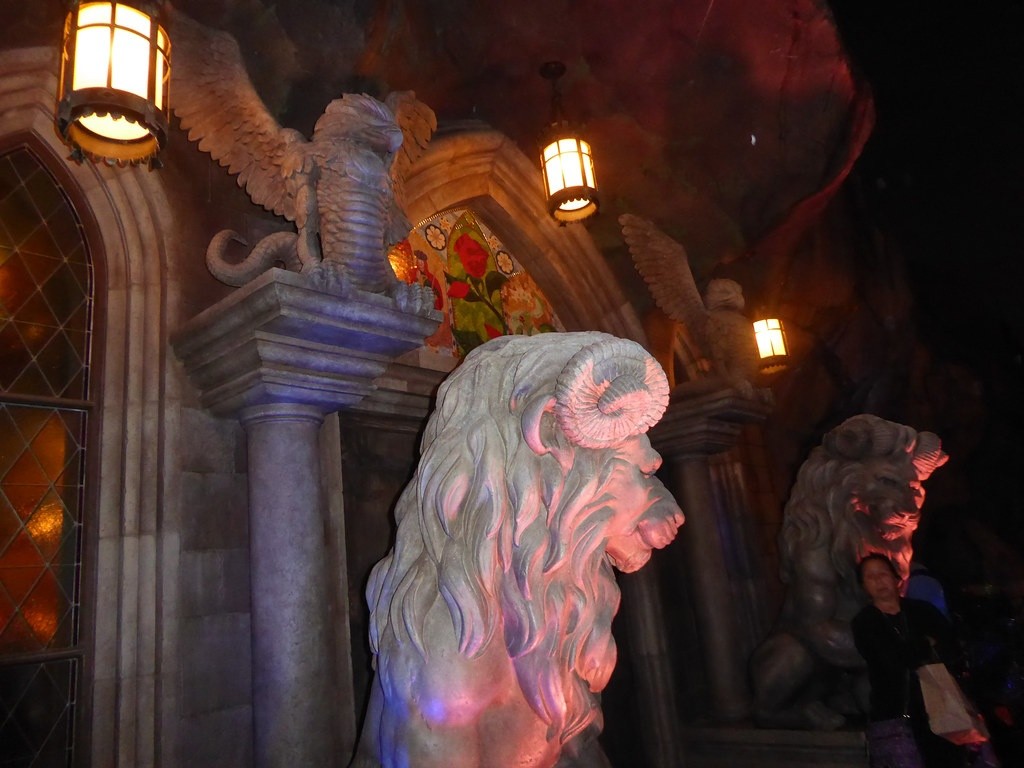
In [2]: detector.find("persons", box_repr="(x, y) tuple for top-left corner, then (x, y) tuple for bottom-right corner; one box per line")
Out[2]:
(852, 554), (980, 768)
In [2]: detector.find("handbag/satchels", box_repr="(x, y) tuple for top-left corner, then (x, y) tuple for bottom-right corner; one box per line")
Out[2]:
(915, 662), (991, 747)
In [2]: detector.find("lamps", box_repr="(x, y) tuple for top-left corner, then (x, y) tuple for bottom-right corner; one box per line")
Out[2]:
(752, 305), (790, 375)
(54, 0), (173, 173)
(539, 59), (601, 226)
